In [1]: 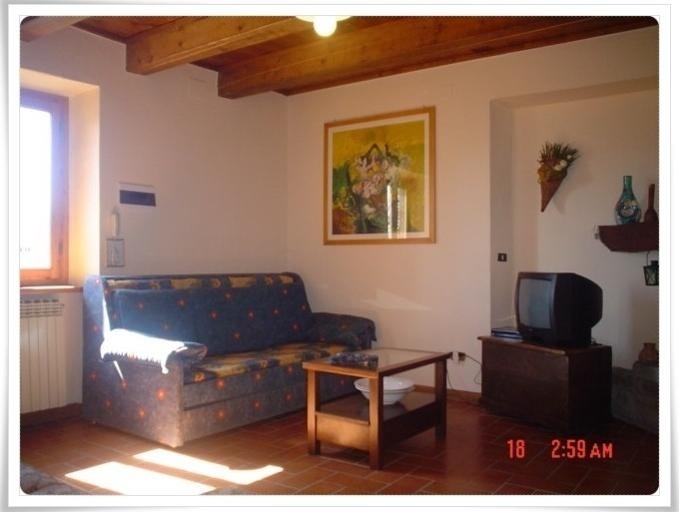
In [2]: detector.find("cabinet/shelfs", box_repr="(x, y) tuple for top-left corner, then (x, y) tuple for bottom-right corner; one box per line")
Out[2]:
(476, 334), (613, 434)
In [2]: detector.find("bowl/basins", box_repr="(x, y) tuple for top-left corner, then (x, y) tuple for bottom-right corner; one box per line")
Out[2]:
(353, 375), (416, 407)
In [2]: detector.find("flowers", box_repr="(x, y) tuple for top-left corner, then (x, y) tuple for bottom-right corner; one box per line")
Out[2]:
(536, 142), (582, 184)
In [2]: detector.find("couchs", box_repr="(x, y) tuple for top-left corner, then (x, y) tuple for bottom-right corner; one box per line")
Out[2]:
(81, 271), (376, 450)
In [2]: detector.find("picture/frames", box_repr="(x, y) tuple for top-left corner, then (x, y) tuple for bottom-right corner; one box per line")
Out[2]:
(322, 104), (437, 247)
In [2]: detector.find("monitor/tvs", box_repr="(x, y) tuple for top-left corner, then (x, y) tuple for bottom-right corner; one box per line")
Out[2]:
(514, 273), (602, 349)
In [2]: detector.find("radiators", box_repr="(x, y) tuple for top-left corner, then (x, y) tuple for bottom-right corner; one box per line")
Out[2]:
(20, 293), (68, 417)
